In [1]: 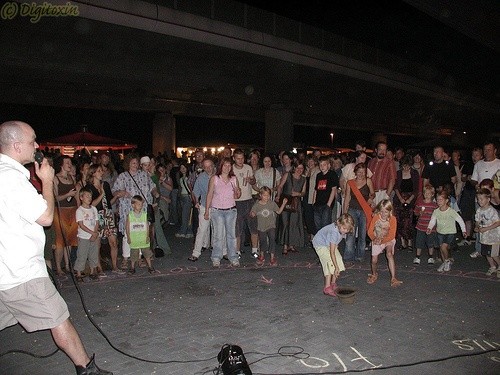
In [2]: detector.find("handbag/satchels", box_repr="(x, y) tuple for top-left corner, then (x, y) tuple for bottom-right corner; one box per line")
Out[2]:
(147, 204), (155, 223)
(278, 193), (298, 211)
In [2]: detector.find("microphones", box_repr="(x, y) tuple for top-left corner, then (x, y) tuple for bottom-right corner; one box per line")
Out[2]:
(35, 151), (45, 165)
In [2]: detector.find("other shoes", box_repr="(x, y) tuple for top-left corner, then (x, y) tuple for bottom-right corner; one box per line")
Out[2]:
(188, 256), (198, 262)
(345, 263), (356, 269)
(413, 257), (455, 266)
(126, 268), (136, 275)
(365, 241), (413, 253)
(76, 273), (97, 281)
(75, 353), (113, 375)
(368, 275), (402, 286)
(456, 238), (472, 247)
(437, 260), (451, 272)
(121, 261), (147, 270)
(174, 234), (194, 238)
(323, 283), (339, 296)
(147, 266), (156, 273)
(56, 268), (76, 277)
(469, 251), (482, 258)
(213, 252), (277, 266)
(486, 265), (500, 278)
(282, 245), (297, 256)
(304, 240), (313, 248)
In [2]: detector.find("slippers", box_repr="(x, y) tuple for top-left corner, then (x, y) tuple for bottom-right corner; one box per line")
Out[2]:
(112, 268), (126, 274)
(97, 272), (107, 278)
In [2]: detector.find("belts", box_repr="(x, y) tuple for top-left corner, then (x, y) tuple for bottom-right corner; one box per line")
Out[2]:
(215, 206), (236, 210)
(374, 187), (388, 192)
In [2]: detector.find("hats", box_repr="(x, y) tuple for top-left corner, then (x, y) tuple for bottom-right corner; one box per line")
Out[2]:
(140, 156), (151, 167)
(334, 287), (360, 303)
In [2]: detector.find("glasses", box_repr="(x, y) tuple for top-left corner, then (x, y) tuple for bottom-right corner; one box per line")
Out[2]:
(195, 155), (203, 158)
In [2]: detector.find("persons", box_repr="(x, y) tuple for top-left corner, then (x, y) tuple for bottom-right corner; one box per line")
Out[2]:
(367, 199), (403, 286)
(313, 214), (354, 299)
(0, 121), (113, 375)
(30, 135), (500, 276)
